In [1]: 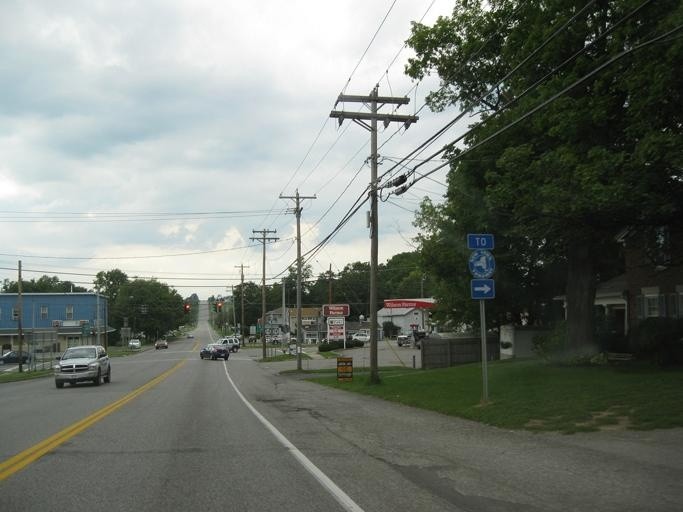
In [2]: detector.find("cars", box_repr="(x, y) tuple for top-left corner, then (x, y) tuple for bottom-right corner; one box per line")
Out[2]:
(0, 351), (31, 366)
(348, 333), (369, 343)
(186, 334), (193, 338)
(154, 339), (168, 349)
(199, 335), (297, 360)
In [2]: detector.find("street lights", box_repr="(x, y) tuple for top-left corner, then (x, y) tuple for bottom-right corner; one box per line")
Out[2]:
(420, 278), (426, 298)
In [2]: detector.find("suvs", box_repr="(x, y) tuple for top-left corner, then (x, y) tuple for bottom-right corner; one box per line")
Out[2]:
(54, 345), (112, 387)
(128, 338), (141, 349)
(396, 329), (412, 346)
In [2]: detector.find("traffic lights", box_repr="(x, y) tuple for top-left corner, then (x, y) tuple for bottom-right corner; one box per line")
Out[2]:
(212, 301), (223, 313)
(184, 304), (191, 313)
